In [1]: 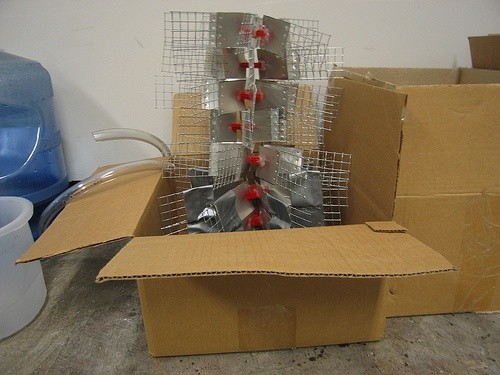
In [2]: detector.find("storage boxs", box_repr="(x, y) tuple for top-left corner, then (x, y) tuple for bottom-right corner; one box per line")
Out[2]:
(15, 75), (458, 358)
(469, 33), (500, 70)
(327, 64), (500, 318)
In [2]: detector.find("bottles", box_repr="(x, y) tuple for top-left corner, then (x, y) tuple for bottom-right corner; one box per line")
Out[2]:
(1, 48), (70, 239)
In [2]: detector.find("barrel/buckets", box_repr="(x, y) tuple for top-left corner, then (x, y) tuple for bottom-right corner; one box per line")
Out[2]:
(1, 195), (51, 341)
